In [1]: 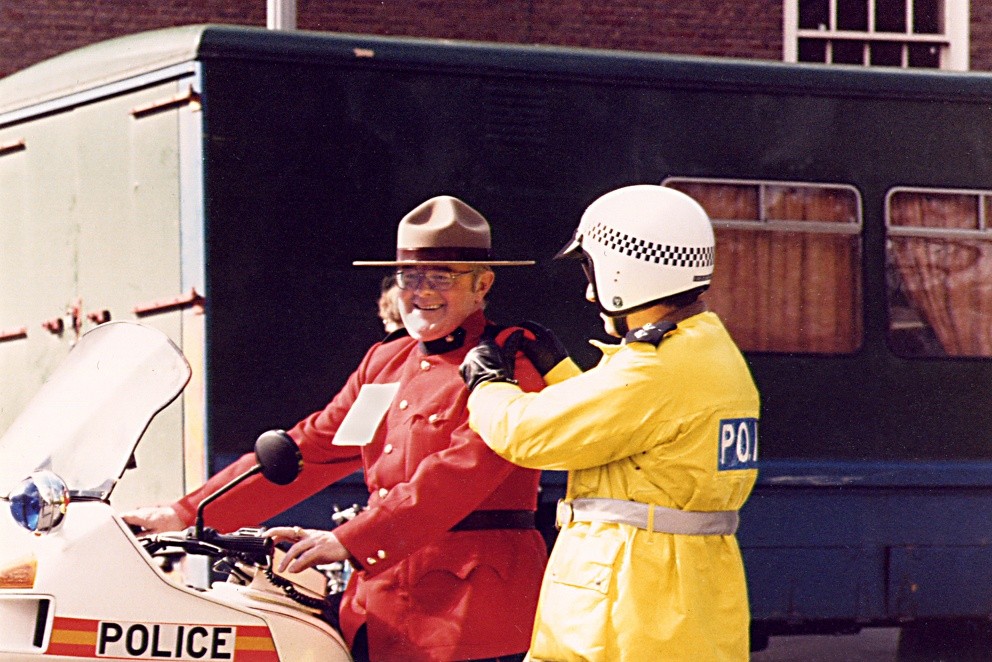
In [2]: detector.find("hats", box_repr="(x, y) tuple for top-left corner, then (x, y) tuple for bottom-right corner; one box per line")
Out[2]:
(352, 196), (536, 266)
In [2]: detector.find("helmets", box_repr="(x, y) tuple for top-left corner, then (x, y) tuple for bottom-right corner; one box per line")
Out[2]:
(552, 185), (714, 317)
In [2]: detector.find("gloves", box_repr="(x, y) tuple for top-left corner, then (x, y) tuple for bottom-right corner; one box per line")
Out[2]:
(519, 319), (569, 374)
(458, 328), (525, 390)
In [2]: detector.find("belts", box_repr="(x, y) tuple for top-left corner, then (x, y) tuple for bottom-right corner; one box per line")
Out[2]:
(554, 496), (740, 535)
(447, 509), (539, 531)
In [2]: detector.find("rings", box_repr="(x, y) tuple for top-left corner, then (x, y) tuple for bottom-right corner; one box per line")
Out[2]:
(293, 525), (301, 538)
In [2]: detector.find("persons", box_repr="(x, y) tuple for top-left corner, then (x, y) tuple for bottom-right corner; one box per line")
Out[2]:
(458, 183), (759, 662)
(120, 195), (548, 662)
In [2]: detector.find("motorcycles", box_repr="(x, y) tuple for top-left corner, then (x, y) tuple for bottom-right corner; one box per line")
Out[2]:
(1, 320), (354, 662)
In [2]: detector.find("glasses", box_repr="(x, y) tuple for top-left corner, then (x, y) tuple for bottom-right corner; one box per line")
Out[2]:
(391, 267), (475, 290)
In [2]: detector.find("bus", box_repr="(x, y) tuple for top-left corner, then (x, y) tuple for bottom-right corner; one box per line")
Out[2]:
(0, 22), (991, 661)
